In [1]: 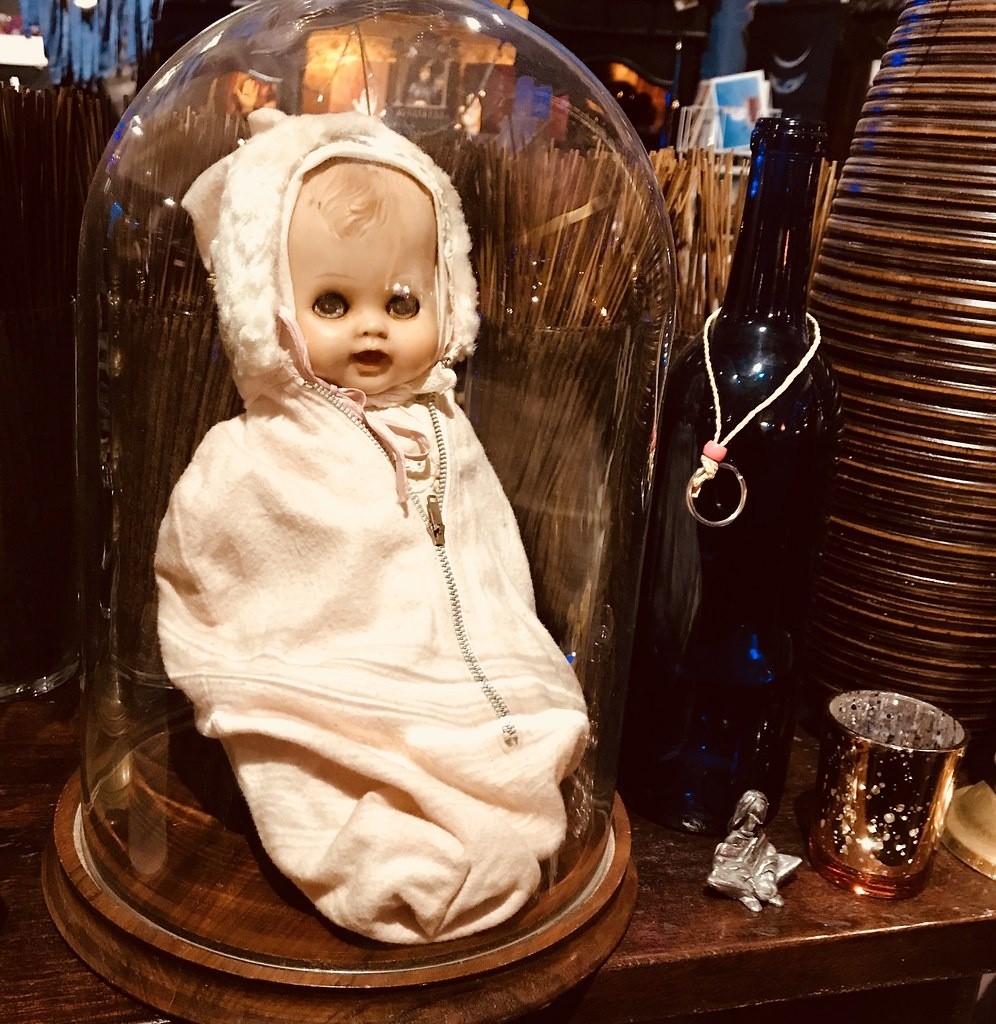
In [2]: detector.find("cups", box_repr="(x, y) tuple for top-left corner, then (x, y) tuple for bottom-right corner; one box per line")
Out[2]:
(809, 688), (968, 900)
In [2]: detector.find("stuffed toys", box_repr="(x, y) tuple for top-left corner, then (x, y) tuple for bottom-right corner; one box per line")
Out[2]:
(153, 107), (589, 944)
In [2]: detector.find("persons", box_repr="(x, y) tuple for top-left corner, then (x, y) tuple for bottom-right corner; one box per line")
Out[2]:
(708, 790), (800, 913)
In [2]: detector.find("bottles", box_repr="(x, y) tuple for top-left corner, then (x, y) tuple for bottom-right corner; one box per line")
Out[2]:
(616, 116), (838, 842)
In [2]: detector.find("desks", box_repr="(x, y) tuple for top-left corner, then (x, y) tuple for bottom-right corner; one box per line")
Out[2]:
(0, 680), (996, 1024)
